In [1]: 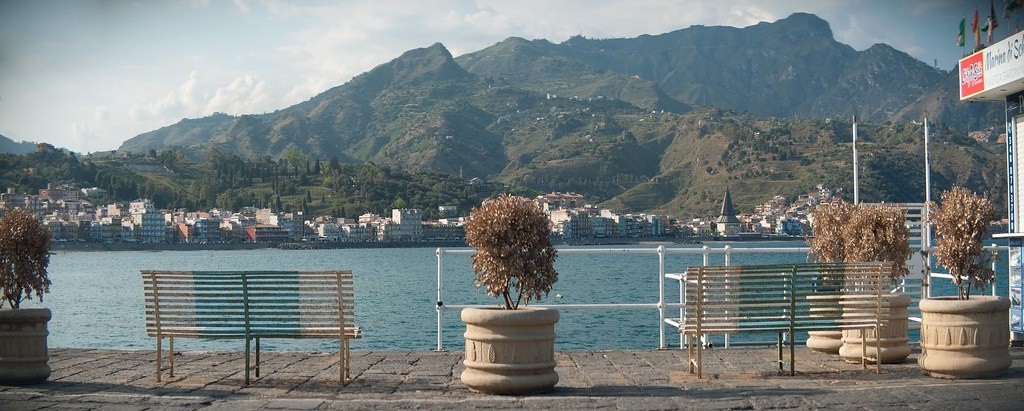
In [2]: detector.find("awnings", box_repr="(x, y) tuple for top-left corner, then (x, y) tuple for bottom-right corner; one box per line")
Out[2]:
(1002, 0), (1013, 20)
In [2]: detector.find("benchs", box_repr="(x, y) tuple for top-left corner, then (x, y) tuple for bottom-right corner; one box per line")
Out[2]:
(675, 259), (896, 379)
(139, 269), (365, 388)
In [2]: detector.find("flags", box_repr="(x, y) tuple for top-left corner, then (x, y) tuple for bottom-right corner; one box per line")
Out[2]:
(980, 0), (999, 45)
(970, 8), (981, 47)
(956, 16), (967, 46)
(1011, 0), (1024, 33)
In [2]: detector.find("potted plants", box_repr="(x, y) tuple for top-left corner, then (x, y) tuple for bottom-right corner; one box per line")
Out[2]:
(0, 205), (57, 387)
(834, 208), (913, 367)
(916, 181), (1013, 379)
(803, 195), (857, 354)
